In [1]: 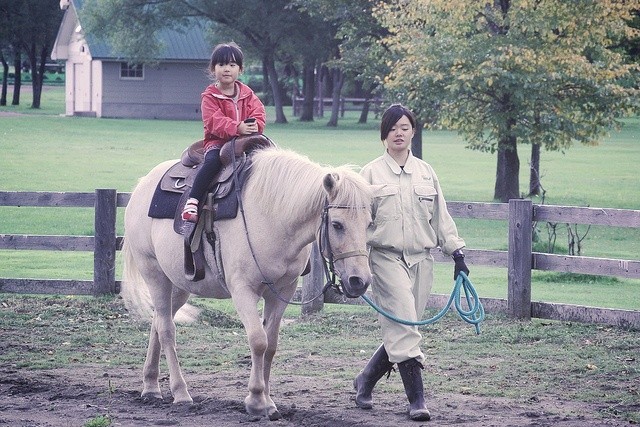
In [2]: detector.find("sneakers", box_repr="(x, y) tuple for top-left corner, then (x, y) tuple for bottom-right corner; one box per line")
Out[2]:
(182, 197), (199, 224)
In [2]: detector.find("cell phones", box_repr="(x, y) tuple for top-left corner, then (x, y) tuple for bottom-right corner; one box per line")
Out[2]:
(244, 118), (260, 123)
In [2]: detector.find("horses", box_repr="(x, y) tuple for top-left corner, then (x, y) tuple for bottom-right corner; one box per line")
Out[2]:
(119, 146), (373, 423)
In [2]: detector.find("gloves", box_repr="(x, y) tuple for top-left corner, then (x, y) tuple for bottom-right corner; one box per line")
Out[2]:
(454, 255), (469, 280)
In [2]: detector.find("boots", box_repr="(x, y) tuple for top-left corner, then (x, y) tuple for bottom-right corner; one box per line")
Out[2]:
(354, 343), (396, 409)
(397, 358), (430, 421)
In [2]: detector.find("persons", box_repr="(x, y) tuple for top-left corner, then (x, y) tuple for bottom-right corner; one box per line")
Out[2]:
(181, 41), (266, 222)
(354, 106), (470, 420)
